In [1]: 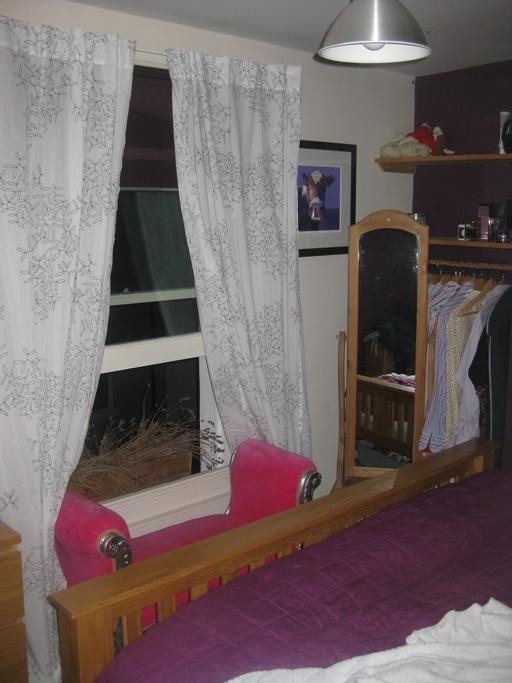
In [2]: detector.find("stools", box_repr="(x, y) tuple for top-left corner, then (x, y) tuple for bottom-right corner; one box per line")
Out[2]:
(53, 439), (322, 633)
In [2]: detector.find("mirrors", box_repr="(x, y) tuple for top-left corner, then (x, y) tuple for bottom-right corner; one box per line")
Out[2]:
(344, 209), (429, 477)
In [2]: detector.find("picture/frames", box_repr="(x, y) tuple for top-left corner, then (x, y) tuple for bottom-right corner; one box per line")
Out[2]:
(297, 139), (357, 257)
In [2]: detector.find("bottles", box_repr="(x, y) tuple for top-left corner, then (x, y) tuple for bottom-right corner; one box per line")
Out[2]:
(470, 216), (481, 241)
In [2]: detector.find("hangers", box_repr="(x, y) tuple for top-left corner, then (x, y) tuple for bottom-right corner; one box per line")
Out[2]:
(428, 256), (512, 317)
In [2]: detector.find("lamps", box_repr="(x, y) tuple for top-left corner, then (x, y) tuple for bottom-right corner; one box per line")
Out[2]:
(316, 0), (432, 67)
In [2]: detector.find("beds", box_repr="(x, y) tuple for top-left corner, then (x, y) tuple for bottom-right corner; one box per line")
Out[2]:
(46, 438), (512, 683)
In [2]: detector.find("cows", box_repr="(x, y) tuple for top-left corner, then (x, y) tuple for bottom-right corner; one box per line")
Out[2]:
(297, 169), (335, 231)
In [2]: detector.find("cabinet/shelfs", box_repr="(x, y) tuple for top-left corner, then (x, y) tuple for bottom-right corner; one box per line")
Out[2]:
(374, 59), (512, 282)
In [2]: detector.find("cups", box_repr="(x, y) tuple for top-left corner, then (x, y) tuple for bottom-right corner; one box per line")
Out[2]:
(458, 224), (471, 240)
